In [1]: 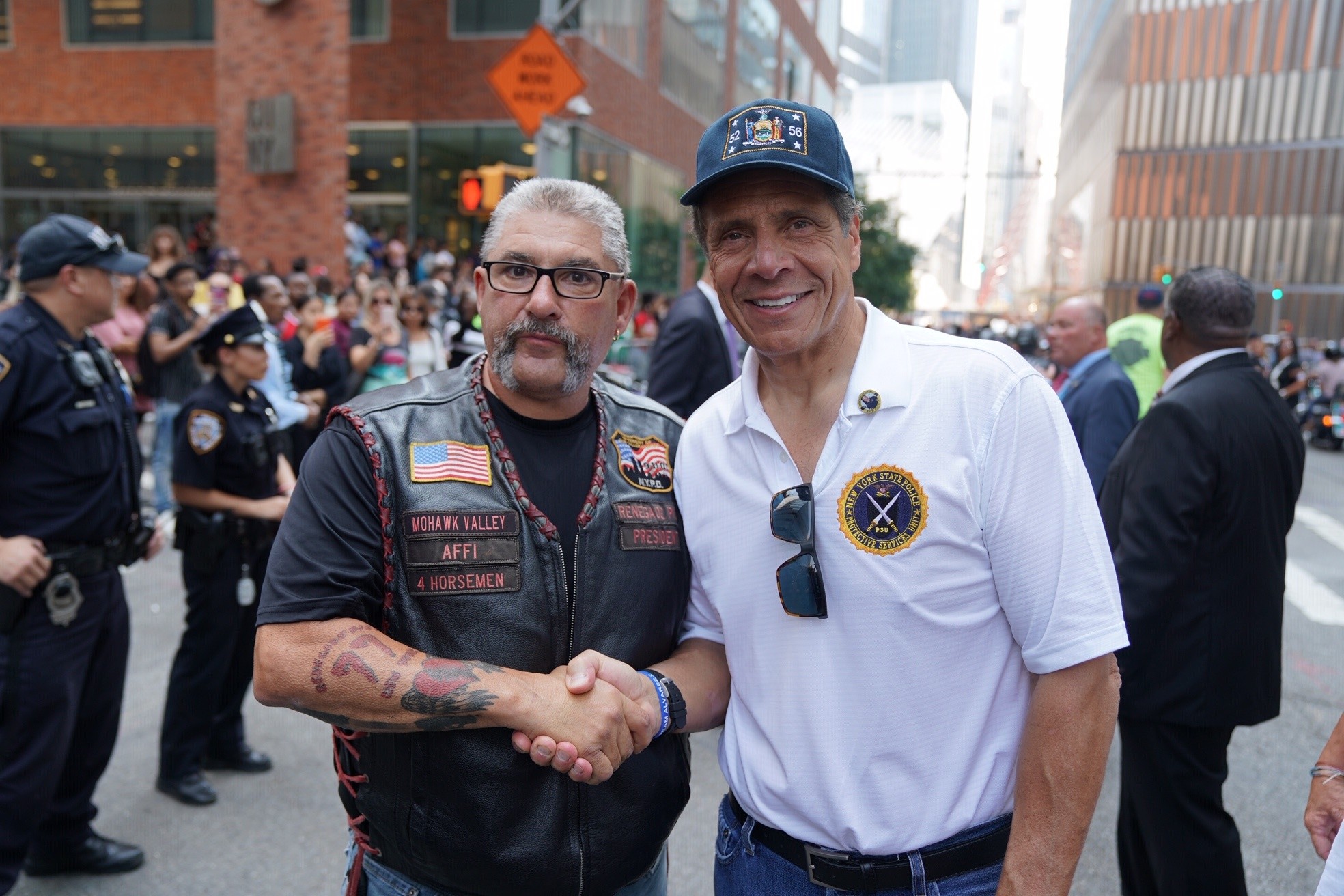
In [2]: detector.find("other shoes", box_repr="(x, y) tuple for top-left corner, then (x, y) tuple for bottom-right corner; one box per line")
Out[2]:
(157, 510), (177, 540)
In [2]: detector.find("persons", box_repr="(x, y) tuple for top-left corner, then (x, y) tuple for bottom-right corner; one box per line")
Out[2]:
(511, 97), (1131, 896)
(1046, 264), (1344, 896)
(597, 260), (755, 422)
(253, 178), (693, 896)
(0, 214), (483, 896)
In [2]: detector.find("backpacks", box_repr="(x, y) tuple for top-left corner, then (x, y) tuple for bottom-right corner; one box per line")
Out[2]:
(132, 302), (179, 399)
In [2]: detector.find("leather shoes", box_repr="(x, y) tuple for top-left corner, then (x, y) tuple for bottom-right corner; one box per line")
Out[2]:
(23, 832), (143, 876)
(156, 768), (218, 805)
(198, 741), (272, 772)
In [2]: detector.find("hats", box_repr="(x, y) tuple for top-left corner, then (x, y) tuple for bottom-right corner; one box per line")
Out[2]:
(16, 212), (151, 281)
(1138, 285), (1164, 309)
(680, 98), (856, 206)
(191, 299), (277, 347)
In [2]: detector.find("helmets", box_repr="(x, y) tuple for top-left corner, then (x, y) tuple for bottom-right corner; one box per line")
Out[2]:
(1325, 344), (1342, 360)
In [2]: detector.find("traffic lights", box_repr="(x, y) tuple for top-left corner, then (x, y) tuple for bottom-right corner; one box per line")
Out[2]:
(481, 168), (527, 214)
(457, 171), (486, 215)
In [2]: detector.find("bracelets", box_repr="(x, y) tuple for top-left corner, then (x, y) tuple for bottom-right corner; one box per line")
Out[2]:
(638, 670), (670, 739)
(645, 669), (687, 735)
(1310, 761), (1344, 784)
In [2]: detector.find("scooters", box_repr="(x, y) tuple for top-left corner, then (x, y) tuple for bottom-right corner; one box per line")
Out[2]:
(1251, 322), (1344, 450)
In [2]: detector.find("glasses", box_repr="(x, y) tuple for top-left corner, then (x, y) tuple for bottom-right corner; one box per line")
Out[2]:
(770, 482), (828, 620)
(371, 298), (391, 305)
(403, 305), (424, 312)
(481, 261), (624, 299)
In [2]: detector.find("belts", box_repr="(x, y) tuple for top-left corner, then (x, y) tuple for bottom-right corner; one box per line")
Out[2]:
(727, 787), (1012, 896)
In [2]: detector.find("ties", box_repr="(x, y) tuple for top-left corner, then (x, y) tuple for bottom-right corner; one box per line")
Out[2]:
(1050, 371), (1069, 393)
(726, 320), (740, 382)
